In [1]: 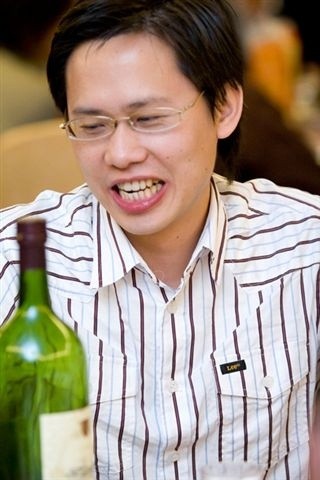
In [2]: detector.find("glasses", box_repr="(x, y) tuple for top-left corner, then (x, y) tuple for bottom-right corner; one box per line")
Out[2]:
(59, 90), (204, 141)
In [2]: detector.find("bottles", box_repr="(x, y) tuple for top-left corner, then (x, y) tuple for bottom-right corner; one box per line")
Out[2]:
(0, 215), (96, 480)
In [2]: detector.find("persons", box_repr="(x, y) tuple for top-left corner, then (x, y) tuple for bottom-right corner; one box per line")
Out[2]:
(0, 0), (320, 480)
